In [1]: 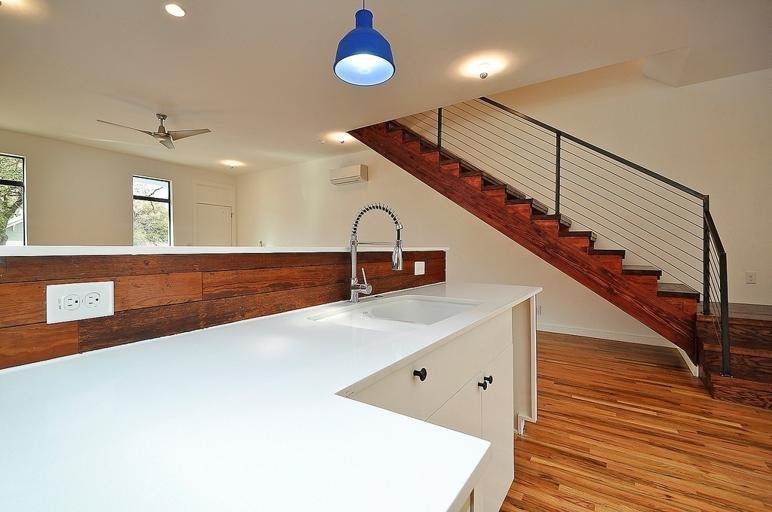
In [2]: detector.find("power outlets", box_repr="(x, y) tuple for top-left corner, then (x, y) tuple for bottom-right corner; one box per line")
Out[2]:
(45, 281), (115, 324)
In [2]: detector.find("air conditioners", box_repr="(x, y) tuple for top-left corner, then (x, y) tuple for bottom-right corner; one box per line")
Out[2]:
(329, 163), (368, 185)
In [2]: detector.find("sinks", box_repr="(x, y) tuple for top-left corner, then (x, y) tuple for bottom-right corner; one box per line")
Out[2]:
(305, 308), (417, 333)
(364, 294), (488, 325)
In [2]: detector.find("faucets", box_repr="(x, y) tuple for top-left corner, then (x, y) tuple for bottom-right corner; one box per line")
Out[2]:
(349, 202), (404, 304)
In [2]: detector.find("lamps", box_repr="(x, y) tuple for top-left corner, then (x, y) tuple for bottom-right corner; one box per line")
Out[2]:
(334, 0), (395, 87)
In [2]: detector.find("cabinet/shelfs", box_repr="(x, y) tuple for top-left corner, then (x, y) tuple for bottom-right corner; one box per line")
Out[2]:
(348, 308), (515, 512)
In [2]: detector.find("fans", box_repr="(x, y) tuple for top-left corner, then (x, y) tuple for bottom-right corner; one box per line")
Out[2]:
(97, 113), (211, 150)
